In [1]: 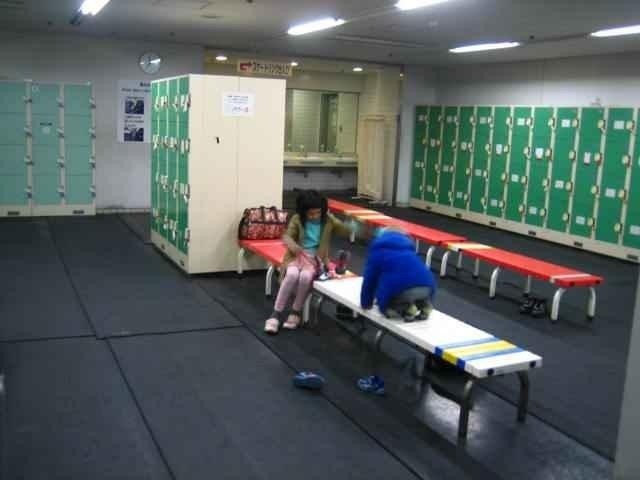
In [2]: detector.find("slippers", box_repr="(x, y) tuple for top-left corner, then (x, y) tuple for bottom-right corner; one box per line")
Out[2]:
(282, 314), (301, 330)
(264, 319), (279, 333)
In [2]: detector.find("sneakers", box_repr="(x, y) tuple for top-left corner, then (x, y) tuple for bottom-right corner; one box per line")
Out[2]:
(530, 294), (548, 318)
(356, 374), (385, 395)
(291, 371), (327, 388)
(518, 291), (537, 313)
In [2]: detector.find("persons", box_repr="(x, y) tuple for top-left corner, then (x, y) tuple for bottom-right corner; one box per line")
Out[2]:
(360, 225), (437, 322)
(262, 188), (355, 335)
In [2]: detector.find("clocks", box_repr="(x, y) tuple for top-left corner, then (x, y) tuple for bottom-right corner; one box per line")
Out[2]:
(136, 48), (163, 76)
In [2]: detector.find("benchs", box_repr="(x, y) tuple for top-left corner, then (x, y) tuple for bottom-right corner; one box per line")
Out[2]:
(326, 199), (382, 243)
(237, 239), (359, 324)
(440, 241), (602, 320)
(312, 277), (542, 435)
(354, 214), (469, 271)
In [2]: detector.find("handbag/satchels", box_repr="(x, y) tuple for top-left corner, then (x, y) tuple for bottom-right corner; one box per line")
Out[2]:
(238, 207), (291, 239)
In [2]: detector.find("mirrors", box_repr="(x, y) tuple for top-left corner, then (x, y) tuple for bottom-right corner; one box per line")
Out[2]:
(284, 86), (361, 157)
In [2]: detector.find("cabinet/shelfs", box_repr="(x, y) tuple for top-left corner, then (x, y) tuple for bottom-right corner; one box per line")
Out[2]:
(408, 101), (640, 266)
(0, 77), (96, 219)
(148, 73), (288, 277)
